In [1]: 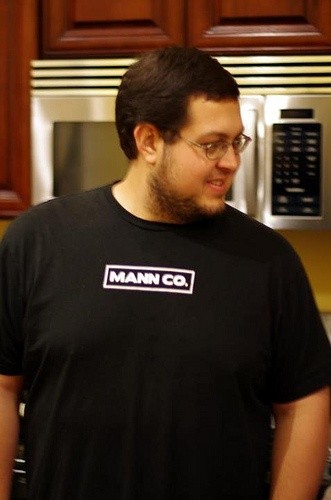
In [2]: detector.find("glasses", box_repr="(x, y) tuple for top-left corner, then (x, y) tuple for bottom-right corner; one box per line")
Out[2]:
(179, 132), (251, 160)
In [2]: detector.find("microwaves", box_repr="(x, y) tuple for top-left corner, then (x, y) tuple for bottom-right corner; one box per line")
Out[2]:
(30, 93), (331, 228)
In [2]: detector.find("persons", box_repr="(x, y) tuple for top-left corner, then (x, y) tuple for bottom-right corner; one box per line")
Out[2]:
(0, 46), (331, 500)
